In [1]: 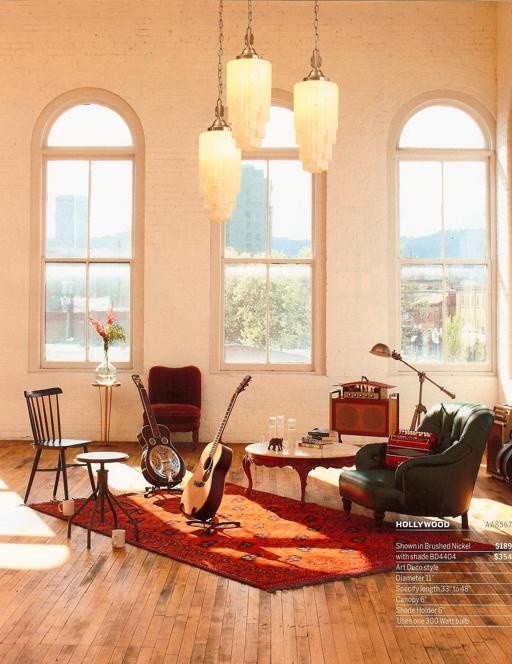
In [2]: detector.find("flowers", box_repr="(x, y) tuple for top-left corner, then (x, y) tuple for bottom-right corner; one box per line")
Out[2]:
(88, 306), (128, 377)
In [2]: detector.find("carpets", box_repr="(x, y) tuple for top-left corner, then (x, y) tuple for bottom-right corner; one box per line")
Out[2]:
(24, 479), (511, 594)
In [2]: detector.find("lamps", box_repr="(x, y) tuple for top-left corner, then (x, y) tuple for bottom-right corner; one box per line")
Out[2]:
(368, 341), (458, 427)
(224, 1), (273, 150)
(292, 0), (342, 177)
(194, 0), (243, 222)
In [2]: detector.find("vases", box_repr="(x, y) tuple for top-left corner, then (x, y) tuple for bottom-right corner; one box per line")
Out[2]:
(95, 349), (118, 386)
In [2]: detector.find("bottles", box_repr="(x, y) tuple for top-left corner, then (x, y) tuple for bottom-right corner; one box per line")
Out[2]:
(269, 415), (297, 453)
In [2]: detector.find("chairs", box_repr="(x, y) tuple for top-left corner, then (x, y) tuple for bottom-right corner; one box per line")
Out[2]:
(142, 364), (203, 444)
(338, 401), (494, 533)
(23, 385), (97, 503)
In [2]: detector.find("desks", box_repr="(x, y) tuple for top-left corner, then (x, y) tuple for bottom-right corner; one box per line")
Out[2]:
(242, 439), (361, 504)
(68, 450), (138, 547)
(91, 382), (121, 443)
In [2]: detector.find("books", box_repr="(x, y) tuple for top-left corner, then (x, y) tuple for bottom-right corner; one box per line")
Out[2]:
(298, 429), (340, 449)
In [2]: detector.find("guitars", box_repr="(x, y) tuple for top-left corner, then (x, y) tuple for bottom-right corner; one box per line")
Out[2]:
(179, 377), (250, 521)
(131, 372), (188, 486)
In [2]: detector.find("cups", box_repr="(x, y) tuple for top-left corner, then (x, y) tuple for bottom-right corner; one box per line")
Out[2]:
(58, 499), (76, 515)
(112, 528), (126, 550)
